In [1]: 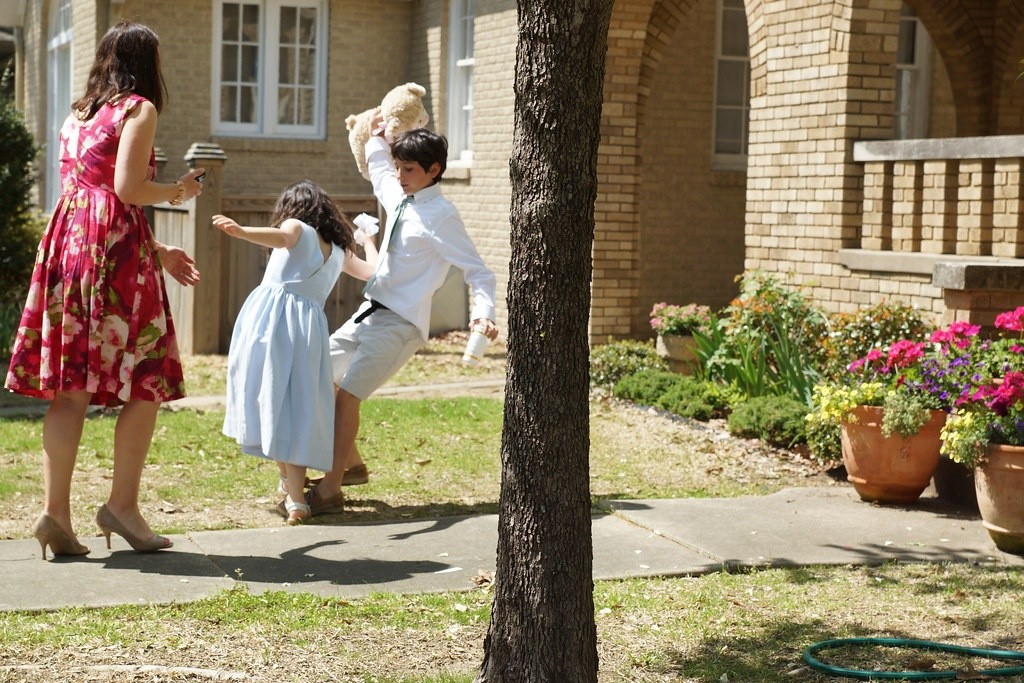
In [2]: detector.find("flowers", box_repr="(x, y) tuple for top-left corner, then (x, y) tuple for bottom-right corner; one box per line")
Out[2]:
(805, 306), (1024, 471)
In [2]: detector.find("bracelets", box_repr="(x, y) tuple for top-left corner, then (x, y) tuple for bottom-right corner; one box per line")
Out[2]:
(170, 180), (187, 207)
(3, 19), (202, 560)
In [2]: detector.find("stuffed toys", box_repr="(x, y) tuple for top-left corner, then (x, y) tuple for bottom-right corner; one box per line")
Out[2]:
(345, 82), (429, 182)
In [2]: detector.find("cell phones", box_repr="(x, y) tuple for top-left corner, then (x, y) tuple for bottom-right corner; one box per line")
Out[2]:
(195, 171), (207, 183)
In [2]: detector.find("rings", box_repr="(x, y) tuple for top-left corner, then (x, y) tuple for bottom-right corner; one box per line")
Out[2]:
(179, 272), (184, 277)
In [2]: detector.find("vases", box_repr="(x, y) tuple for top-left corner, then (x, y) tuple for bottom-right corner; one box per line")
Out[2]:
(834, 404), (949, 505)
(969, 440), (1024, 556)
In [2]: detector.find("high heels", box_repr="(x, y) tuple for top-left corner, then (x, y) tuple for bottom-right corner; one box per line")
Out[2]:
(32, 515), (92, 562)
(95, 505), (175, 549)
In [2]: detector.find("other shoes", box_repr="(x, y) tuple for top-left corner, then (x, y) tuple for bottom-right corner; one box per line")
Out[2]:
(278, 474), (314, 519)
(311, 464), (370, 486)
(278, 487), (344, 516)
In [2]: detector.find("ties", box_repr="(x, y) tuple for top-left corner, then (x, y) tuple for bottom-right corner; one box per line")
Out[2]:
(360, 197), (410, 296)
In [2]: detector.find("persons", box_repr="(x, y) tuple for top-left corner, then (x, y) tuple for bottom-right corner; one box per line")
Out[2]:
(274, 118), (498, 514)
(211, 180), (380, 527)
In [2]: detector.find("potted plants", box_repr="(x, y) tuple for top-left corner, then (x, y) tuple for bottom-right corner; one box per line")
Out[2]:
(588, 290), (838, 464)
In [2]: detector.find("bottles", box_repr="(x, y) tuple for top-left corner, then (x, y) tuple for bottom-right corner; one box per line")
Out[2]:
(462, 325), (490, 368)
